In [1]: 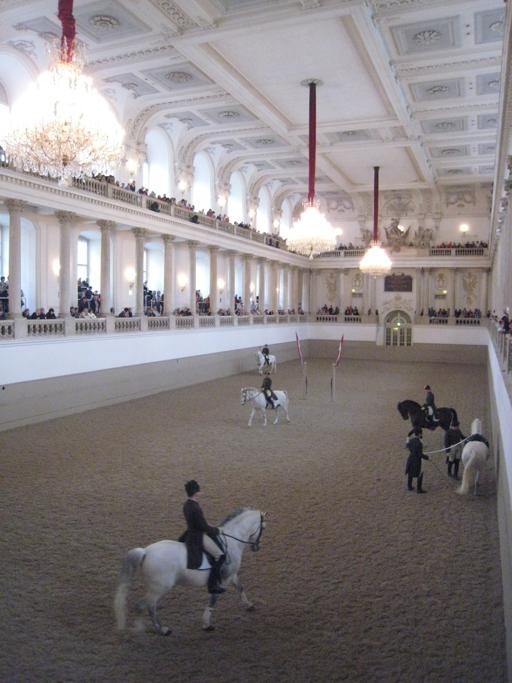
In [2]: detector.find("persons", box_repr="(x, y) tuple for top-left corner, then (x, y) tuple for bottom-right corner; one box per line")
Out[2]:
(177, 479), (229, 595)
(337, 243), (360, 256)
(404, 427), (432, 494)
(422, 307), (480, 325)
(443, 419), (468, 480)
(253, 343), (279, 409)
(1, 275), (261, 335)
(487, 310), (511, 335)
(75, 170), (287, 248)
(432, 241), (487, 255)
(265, 304), (378, 321)
(420, 383), (441, 426)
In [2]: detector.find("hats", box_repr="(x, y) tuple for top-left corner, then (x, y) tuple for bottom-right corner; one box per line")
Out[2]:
(185, 480), (201, 497)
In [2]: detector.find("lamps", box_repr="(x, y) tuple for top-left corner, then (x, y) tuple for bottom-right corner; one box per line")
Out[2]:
(0, 0), (128, 180)
(286, 77), (344, 259)
(358, 166), (393, 277)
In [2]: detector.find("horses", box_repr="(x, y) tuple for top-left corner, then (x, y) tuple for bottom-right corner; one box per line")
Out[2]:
(240, 386), (291, 429)
(397, 399), (458, 449)
(254, 347), (277, 376)
(455, 418), (489, 497)
(113, 504), (270, 637)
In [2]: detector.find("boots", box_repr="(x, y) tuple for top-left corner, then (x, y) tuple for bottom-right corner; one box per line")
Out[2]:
(208, 554), (226, 595)
